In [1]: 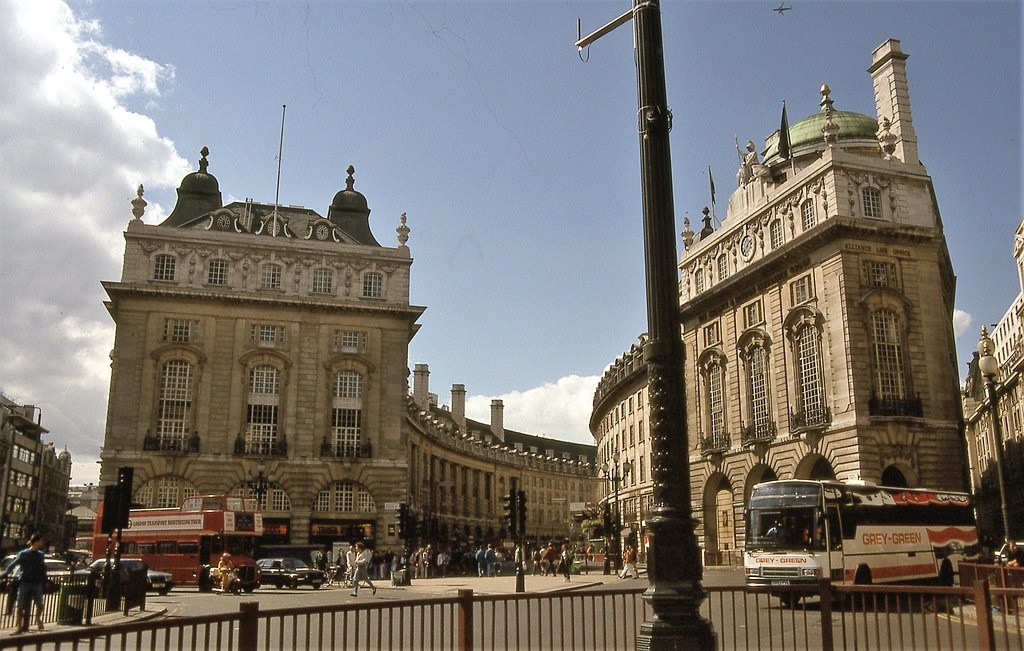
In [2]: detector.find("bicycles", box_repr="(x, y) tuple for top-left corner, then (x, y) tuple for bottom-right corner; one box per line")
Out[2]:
(322, 562), (365, 588)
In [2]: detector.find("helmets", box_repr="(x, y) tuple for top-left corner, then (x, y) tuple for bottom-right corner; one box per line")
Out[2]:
(222, 553), (229, 561)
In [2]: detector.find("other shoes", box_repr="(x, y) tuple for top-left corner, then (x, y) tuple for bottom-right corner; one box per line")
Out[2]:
(36, 620), (44, 631)
(9, 630), (22, 636)
(350, 594), (357, 597)
(372, 587), (376, 595)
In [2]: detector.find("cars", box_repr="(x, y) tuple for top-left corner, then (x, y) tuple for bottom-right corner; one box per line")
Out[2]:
(75, 558), (175, 596)
(0, 548), (91, 581)
(256, 558), (326, 590)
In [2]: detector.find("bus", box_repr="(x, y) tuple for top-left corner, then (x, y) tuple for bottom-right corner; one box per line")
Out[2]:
(744, 474), (982, 608)
(92, 495), (262, 594)
(75, 537), (93, 550)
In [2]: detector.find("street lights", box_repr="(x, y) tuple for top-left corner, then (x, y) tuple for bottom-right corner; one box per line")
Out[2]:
(601, 447), (632, 569)
(976, 324), (1010, 564)
(246, 459), (276, 558)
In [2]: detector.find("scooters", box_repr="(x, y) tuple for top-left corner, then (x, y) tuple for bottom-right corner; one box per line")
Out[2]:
(209, 566), (244, 596)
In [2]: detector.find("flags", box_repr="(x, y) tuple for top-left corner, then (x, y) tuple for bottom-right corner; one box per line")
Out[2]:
(779, 106), (788, 160)
(709, 168), (716, 204)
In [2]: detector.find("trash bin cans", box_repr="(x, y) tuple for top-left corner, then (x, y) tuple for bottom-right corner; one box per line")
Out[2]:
(391, 571), (405, 586)
(57, 582), (85, 620)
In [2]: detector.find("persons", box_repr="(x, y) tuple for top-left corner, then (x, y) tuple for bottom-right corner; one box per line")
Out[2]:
(217, 553), (232, 590)
(980, 534), (1024, 613)
(767, 519), (785, 539)
(315, 541), (639, 597)
(0, 532), (89, 636)
(737, 142), (758, 187)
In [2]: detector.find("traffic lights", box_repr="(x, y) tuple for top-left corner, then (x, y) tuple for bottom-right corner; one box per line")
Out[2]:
(394, 502), (420, 540)
(503, 490), (514, 525)
(516, 489), (527, 526)
(595, 507), (610, 536)
